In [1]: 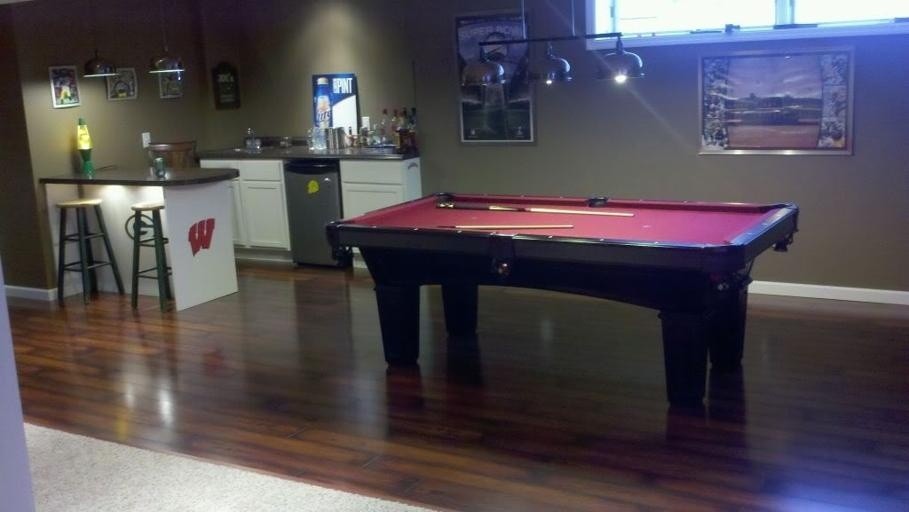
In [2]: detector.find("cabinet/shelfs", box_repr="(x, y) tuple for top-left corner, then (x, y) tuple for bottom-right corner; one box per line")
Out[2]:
(337, 158), (422, 270)
(201, 159), (291, 254)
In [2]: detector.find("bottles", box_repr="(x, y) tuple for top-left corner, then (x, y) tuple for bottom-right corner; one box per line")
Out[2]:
(346, 126), (354, 147)
(382, 107), (416, 147)
(315, 78), (332, 127)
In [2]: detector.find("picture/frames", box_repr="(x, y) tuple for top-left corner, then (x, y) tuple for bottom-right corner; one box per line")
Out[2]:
(452, 7), (536, 144)
(48, 59), (240, 110)
(697, 45), (854, 156)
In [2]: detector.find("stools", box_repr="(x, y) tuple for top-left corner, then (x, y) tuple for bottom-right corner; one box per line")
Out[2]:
(55, 199), (173, 314)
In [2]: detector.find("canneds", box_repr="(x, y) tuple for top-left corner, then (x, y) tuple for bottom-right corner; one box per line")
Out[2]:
(153, 157), (165, 178)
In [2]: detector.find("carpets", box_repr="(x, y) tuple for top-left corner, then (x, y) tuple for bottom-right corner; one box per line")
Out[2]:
(21, 420), (438, 512)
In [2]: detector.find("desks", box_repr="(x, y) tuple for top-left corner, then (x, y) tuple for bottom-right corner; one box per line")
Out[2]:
(322, 191), (800, 404)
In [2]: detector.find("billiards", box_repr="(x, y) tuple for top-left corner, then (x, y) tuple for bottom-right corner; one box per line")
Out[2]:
(499, 264), (509, 274)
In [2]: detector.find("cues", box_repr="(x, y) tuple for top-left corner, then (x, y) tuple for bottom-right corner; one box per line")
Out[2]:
(437, 225), (573, 229)
(437, 202), (634, 217)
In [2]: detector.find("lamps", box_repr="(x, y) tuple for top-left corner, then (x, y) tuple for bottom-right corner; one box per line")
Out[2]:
(83, 1), (119, 78)
(147, 0), (186, 74)
(461, 0), (646, 88)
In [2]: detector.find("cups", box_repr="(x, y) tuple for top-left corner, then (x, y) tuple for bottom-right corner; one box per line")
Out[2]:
(154, 158), (165, 176)
